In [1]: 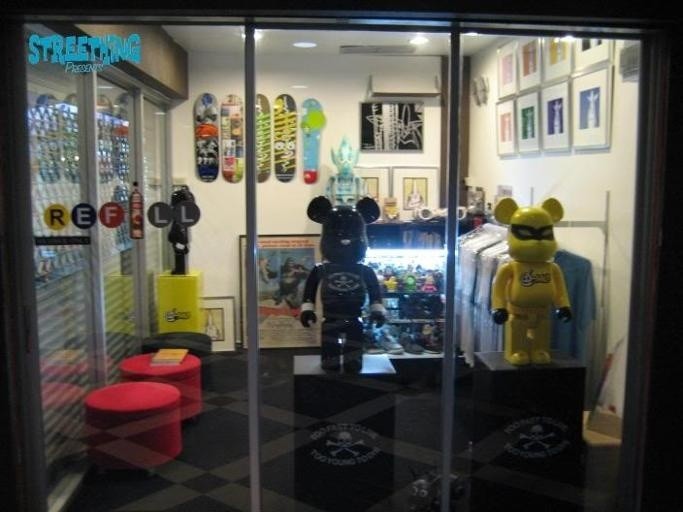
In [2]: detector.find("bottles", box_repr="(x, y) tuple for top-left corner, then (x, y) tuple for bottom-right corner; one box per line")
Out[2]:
(129, 180), (145, 239)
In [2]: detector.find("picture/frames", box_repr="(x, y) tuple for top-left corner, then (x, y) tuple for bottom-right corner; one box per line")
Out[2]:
(493, 35), (615, 160)
(352, 164), (440, 221)
(198, 234), (327, 355)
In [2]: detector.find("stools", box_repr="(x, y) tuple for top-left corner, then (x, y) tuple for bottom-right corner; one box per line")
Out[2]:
(80, 331), (211, 478)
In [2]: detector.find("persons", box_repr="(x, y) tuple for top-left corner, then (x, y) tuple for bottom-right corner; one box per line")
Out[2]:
(325, 137), (364, 207)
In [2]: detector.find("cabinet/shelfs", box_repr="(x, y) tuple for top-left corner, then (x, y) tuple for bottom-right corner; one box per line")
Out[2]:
(358, 290), (446, 359)
